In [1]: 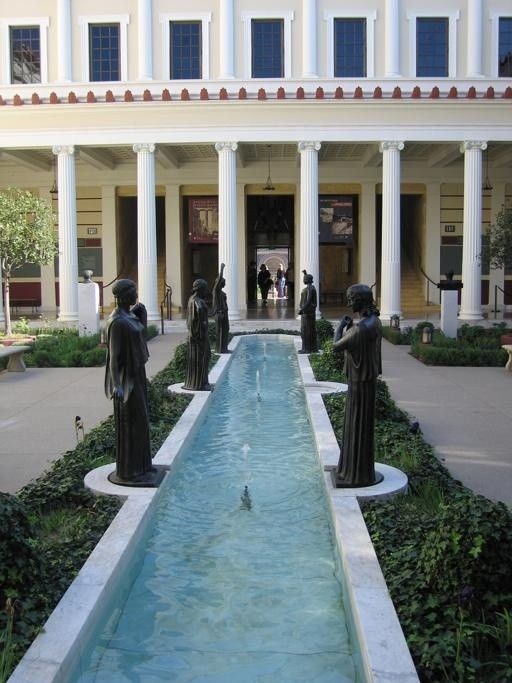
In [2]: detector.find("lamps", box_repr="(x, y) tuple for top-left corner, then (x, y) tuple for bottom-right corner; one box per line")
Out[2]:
(262, 144), (275, 190)
(422, 327), (432, 344)
(482, 145), (493, 190)
(391, 314), (400, 328)
(49, 154), (57, 193)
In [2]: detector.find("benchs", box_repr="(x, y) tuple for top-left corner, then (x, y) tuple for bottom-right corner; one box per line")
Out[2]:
(0, 345), (30, 372)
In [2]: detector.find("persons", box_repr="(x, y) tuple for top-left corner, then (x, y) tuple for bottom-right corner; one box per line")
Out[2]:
(185, 280), (213, 388)
(213, 263), (229, 354)
(297, 270), (318, 354)
(104, 280), (155, 479)
(332, 283), (384, 486)
(286, 263), (294, 301)
(248, 261), (257, 302)
(258, 264), (271, 303)
(275, 263), (287, 299)
